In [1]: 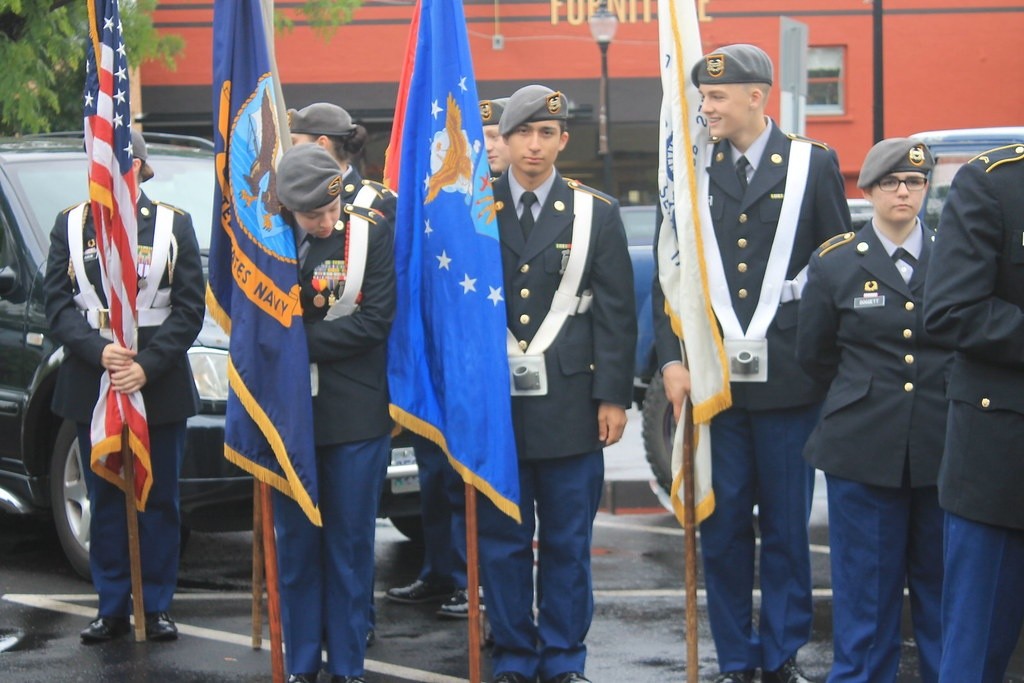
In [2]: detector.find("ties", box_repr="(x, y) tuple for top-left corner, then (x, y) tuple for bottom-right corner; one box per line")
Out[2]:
(519, 191), (538, 243)
(736, 155), (751, 196)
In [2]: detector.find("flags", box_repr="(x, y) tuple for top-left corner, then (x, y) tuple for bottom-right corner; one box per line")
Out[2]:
(206, 0), (324, 527)
(382, 1), (523, 525)
(656, 0), (731, 527)
(82, 0), (153, 512)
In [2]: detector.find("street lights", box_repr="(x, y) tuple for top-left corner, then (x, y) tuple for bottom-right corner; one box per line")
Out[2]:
(589, 0), (618, 153)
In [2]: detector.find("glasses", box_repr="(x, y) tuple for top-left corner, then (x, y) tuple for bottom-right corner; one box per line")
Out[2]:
(872, 175), (927, 192)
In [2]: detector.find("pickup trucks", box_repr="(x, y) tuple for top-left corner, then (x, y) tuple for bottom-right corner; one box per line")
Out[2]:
(621, 128), (1023, 514)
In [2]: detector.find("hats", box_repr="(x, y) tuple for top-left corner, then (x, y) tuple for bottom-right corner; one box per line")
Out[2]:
(83, 128), (148, 162)
(857, 136), (936, 189)
(478, 97), (510, 126)
(277, 143), (344, 212)
(287, 102), (357, 136)
(499, 85), (568, 136)
(691, 44), (773, 89)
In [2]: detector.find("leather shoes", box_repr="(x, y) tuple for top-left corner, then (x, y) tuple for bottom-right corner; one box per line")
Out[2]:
(365, 630), (376, 647)
(80, 615), (131, 640)
(712, 658), (812, 683)
(287, 672), (368, 683)
(384, 580), (488, 619)
(492, 670), (592, 683)
(145, 612), (178, 640)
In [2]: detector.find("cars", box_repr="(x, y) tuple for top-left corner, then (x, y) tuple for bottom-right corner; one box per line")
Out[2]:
(0, 130), (425, 585)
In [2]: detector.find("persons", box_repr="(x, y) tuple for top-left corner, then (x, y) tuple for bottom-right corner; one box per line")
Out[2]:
(795, 138), (955, 683)
(276, 103), (399, 683)
(921, 144), (1024, 683)
(43, 128), (206, 643)
(650, 45), (853, 683)
(386, 85), (639, 683)
